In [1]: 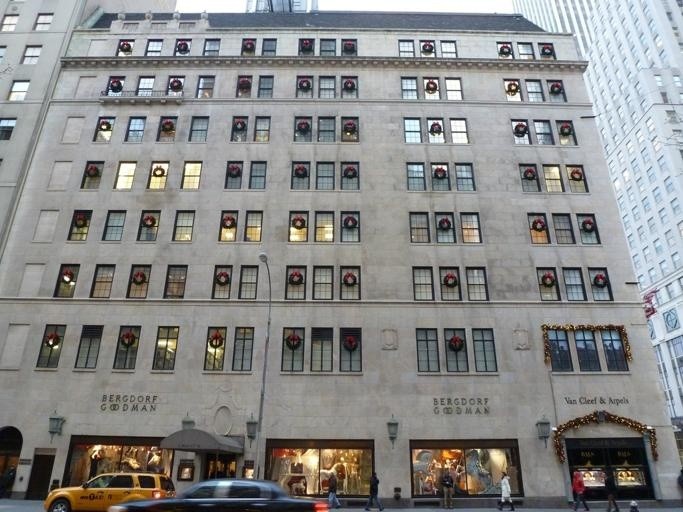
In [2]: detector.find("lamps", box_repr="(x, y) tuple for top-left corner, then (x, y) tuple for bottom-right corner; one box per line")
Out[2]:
(180, 409), (260, 449)
(48, 408), (66, 446)
(387, 412), (401, 448)
(535, 414), (552, 450)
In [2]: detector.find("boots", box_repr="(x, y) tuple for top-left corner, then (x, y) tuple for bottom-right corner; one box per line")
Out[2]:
(498, 500), (516, 511)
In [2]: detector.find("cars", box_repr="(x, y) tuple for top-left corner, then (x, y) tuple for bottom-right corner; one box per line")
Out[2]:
(44, 472), (176, 511)
(106, 477), (328, 512)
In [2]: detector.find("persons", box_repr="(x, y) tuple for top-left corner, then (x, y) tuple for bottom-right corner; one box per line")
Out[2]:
(572, 471), (589, 511)
(1, 463), (16, 498)
(602, 465), (620, 512)
(98, 446), (164, 473)
(328, 472), (341, 509)
(87, 449), (102, 481)
(440, 469), (453, 509)
(210, 466), (226, 479)
(413, 449), (496, 496)
(496, 470), (515, 511)
(629, 498), (640, 512)
(364, 472), (382, 511)
(277, 456), (362, 494)
(677, 469), (683, 487)
